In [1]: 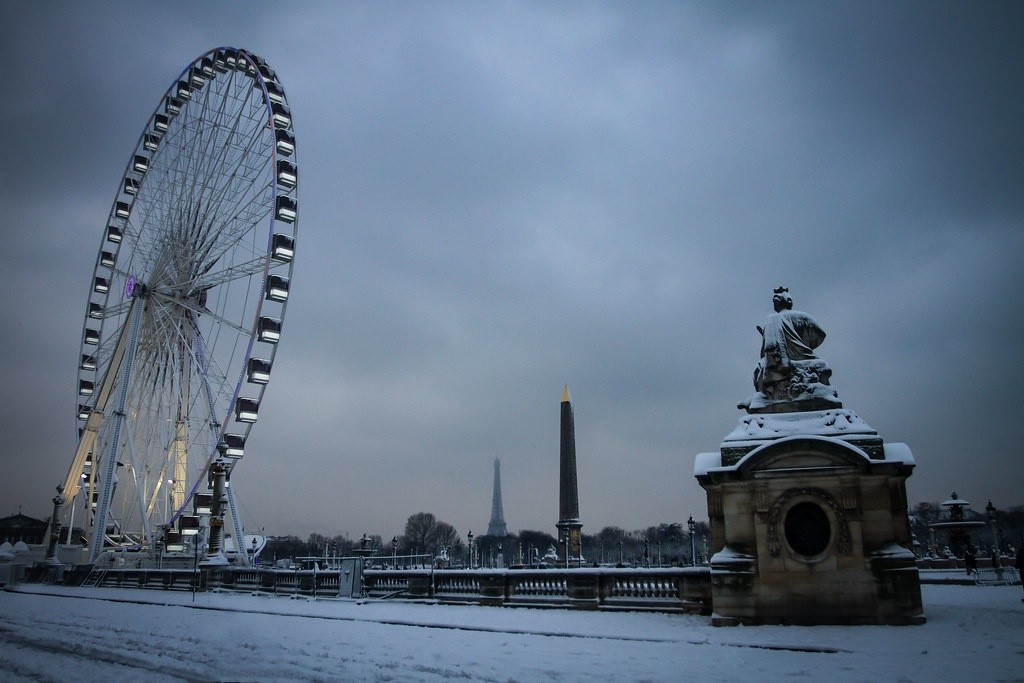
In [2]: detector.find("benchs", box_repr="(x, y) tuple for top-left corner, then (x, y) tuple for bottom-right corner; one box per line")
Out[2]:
(972, 567), (1021, 586)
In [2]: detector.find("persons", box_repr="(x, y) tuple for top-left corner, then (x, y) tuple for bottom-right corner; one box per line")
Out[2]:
(753, 287), (833, 391)
(1014, 548), (1024, 601)
(965, 550), (978, 575)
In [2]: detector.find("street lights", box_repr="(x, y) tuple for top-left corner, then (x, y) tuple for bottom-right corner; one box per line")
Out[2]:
(251, 538), (257, 566)
(687, 513), (699, 566)
(986, 499), (1004, 581)
(392, 536), (399, 569)
(467, 529), (474, 569)
(562, 524), (571, 568)
